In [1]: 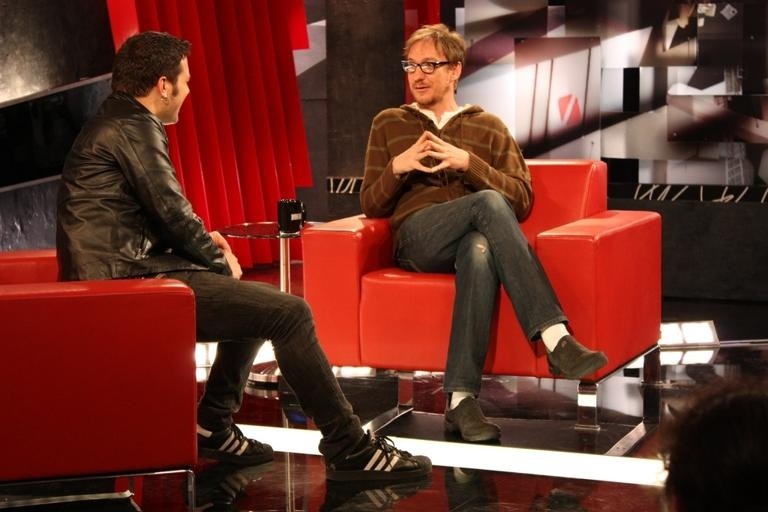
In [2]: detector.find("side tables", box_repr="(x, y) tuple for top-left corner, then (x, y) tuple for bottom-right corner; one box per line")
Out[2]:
(218, 219), (328, 388)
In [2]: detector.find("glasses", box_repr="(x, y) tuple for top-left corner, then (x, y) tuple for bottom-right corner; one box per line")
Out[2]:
(400, 60), (450, 74)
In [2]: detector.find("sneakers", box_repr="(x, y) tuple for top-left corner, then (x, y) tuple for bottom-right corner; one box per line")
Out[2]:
(443, 392), (501, 441)
(326, 429), (432, 481)
(446, 468), (484, 512)
(197, 422), (273, 465)
(180, 461), (273, 512)
(547, 335), (608, 380)
(326, 480), (432, 512)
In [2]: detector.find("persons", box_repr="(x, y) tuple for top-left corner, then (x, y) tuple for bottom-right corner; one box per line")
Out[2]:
(54, 32), (434, 483)
(649, 356), (767, 512)
(184, 461), (424, 511)
(445, 467), (580, 512)
(359, 20), (609, 442)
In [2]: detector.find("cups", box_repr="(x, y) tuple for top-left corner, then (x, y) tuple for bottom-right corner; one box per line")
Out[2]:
(277, 198), (307, 233)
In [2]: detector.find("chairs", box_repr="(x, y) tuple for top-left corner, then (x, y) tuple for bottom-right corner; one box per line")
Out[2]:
(300, 156), (663, 432)
(0, 244), (198, 507)
